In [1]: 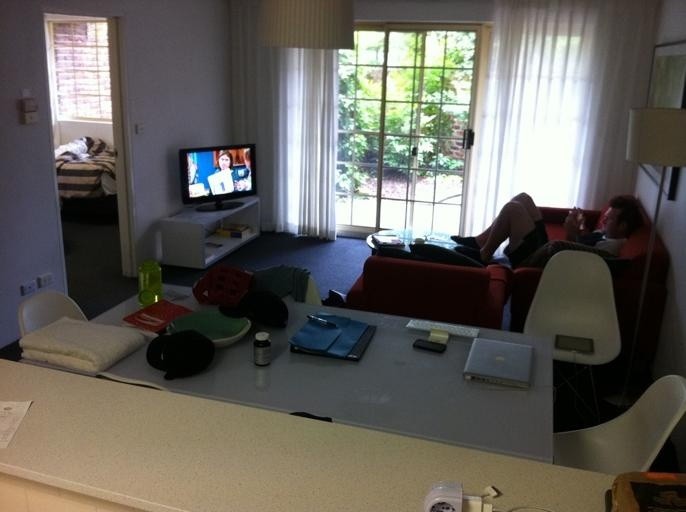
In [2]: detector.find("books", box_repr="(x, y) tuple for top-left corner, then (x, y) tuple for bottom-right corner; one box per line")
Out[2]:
(122, 299), (192, 335)
(372, 235), (405, 249)
(291, 312), (378, 361)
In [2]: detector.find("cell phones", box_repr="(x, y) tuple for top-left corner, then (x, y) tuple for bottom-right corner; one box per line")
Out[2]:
(413, 339), (447, 353)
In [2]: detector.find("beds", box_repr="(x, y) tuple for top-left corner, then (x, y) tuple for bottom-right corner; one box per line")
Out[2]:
(55, 147), (118, 226)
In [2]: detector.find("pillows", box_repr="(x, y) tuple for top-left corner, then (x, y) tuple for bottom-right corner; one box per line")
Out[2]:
(410, 244), (484, 268)
(520, 240), (615, 269)
(381, 247), (412, 258)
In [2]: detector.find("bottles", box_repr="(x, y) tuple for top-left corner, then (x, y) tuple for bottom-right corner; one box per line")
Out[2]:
(138, 259), (163, 308)
(253, 331), (272, 366)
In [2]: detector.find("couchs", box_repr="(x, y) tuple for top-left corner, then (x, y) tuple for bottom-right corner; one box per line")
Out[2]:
(509, 198), (670, 390)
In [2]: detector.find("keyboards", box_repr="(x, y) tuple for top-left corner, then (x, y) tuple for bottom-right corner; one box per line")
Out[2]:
(406, 320), (480, 338)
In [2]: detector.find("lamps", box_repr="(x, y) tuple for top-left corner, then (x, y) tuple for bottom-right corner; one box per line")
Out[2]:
(256, 0), (354, 51)
(627, 106), (686, 168)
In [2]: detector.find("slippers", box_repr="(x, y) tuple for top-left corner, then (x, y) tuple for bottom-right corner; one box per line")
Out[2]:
(451, 235), (485, 266)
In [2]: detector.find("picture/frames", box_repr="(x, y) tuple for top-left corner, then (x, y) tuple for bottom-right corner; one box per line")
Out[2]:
(640, 39), (686, 199)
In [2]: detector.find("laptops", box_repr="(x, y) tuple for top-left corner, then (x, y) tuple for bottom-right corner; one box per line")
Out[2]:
(463, 339), (533, 389)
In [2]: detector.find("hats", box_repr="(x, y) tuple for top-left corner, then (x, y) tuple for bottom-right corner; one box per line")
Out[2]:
(145, 328), (216, 381)
(219, 288), (289, 329)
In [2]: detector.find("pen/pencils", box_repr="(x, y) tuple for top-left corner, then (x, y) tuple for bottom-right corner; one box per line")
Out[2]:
(307, 314), (336, 327)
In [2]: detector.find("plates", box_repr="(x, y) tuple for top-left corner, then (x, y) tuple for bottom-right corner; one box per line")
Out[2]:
(166, 313), (250, 348)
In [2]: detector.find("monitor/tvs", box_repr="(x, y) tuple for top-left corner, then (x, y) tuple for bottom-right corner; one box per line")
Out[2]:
(181, 144), (258, 212)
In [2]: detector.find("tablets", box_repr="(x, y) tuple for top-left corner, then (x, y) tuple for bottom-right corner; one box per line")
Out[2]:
(555, 335), (594, 355)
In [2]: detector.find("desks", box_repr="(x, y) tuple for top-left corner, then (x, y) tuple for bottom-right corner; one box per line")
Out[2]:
(17, 283), (554, 465)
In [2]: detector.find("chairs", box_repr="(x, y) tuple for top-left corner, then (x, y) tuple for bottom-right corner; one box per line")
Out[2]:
(347, 256), (515, 329)
(554, 374), (685, 476)
(18, 288), (89, 338)
(528, 249), (623, 423)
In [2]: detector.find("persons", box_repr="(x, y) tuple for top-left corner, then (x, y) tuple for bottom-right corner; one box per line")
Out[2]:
(234, 148), (253, 192)
(451, 192), (643, 270)
(208, 151), (240, 196)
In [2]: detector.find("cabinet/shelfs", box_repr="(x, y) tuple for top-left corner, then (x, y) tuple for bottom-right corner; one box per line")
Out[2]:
(161, 195), (262, 272)
